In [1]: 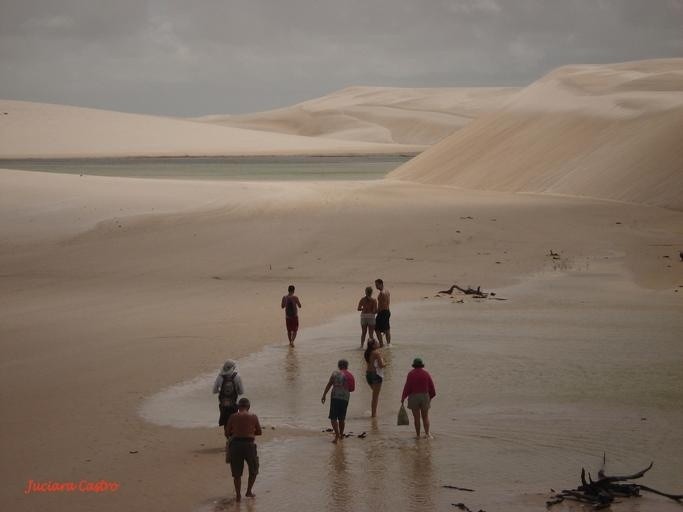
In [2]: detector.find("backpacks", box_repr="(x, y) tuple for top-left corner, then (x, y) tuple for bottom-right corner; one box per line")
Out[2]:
(332, 370), (348, 400)
(219, 372), (237, 405)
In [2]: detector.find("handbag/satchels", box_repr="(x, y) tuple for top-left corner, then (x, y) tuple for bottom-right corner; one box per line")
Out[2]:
(398, 406), (409, 425)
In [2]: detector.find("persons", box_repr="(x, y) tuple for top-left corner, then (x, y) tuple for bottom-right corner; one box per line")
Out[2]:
(401, 358), (436, 439)
(357, 286), (377, 349)
(212, 358), (244, 426)
(375, 279), (391, 348)
(281, 285), (301, 347)
(364, 337), (387, 417)
(225, 398), (262, 502)
(321, 359), (355, 443)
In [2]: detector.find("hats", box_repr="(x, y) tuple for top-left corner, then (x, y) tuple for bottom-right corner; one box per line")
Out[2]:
(412, 358), (424, 367)
(220, 360), (235, 375)
